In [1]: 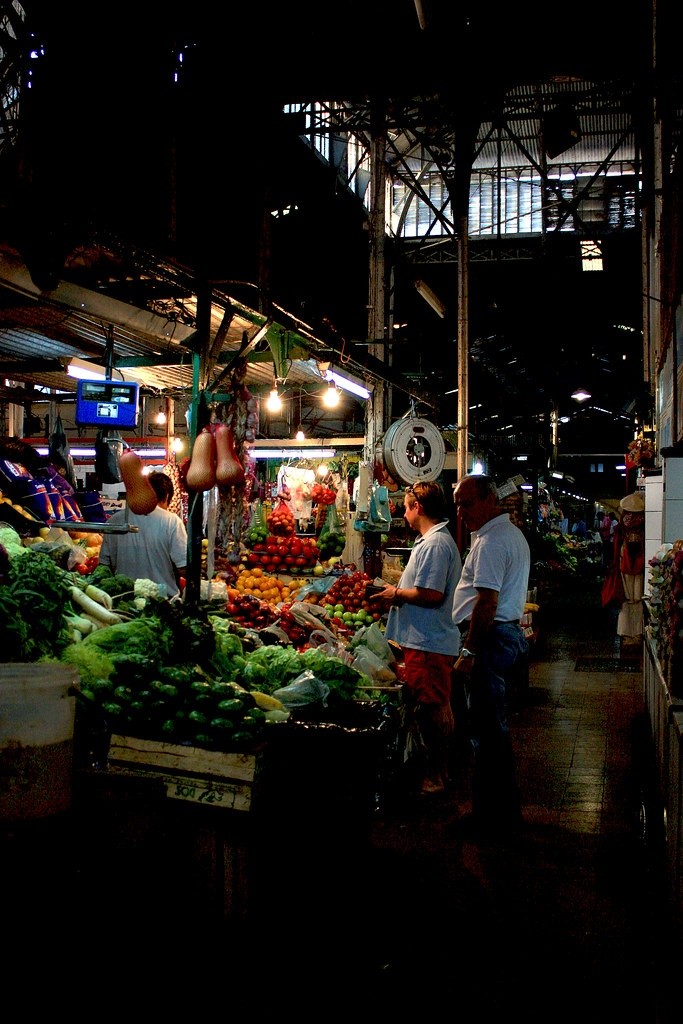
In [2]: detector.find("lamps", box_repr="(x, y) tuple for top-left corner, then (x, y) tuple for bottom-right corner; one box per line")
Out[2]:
(171, 432), (188, 451)
(413, 279), (446, 317)
(157, 405), (172, 424)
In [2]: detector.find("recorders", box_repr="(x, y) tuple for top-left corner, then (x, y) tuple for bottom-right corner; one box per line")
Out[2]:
(75, 379), (139, 430)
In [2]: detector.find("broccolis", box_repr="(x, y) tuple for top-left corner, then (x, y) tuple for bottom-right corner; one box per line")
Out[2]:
(90, 564), (153, 614)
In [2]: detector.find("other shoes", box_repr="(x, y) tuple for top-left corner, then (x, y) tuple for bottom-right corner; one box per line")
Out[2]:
(446, 817), (523, 845)
(400, 784), (449, 806)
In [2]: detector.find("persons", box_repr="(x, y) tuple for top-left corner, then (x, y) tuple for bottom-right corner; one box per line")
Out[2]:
(609, 512), (618, 560)
(99, 471), (187, 597)
(572, 512), (588, 537)
(552, 510), (568, 534)
(448, 475), (530, 838)
(369, 482), (462, 793)
(596, 512), (612, 554)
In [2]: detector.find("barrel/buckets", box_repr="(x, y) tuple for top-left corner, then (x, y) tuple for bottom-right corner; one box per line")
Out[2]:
(0, 663), (112, 822)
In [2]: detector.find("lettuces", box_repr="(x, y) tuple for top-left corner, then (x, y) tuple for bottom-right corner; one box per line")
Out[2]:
(80, 615), (383, 702)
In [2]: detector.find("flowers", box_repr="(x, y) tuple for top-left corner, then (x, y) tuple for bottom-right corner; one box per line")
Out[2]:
(627, 437), (656, 467)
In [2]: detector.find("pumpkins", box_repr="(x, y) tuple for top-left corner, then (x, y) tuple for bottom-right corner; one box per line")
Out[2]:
(185, 428), (217, 492)
(214, 425), (246, 485)
(119, 450), (157, 517)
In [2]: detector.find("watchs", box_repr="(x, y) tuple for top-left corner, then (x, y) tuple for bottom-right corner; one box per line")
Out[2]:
(461, 648), (476, 658)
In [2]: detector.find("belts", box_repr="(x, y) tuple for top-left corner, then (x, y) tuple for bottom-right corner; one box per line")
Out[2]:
(457, 620), (470, 633)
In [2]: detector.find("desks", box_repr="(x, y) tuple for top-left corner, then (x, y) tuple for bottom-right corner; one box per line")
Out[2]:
(643, 624), (682, 935)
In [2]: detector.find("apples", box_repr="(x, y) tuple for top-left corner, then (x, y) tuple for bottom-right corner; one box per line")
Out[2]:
(300, 570), (391, 628)
(222, 595), (329, 654)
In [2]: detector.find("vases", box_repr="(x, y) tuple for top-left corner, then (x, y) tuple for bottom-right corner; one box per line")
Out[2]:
(642, 467), (668, 481)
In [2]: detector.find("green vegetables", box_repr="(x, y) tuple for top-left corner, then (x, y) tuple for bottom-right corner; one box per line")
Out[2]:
(0, 550), (77, 660)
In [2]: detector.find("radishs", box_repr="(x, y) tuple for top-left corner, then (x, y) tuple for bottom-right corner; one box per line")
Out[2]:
(63, 583), (124, 641)
